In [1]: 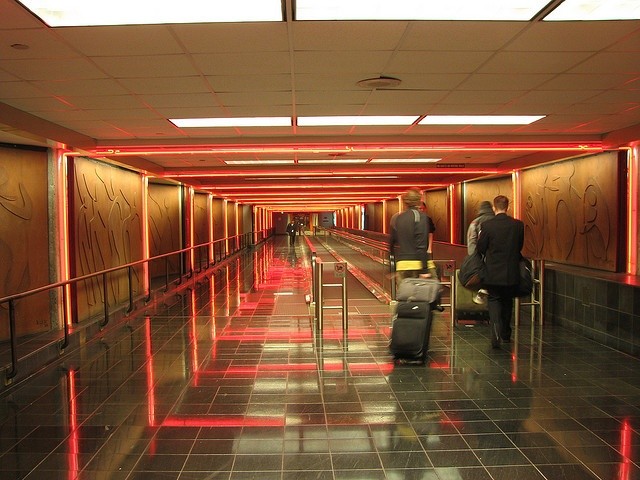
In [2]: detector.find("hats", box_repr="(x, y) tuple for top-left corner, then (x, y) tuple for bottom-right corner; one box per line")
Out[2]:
(477, 201), (494, 215)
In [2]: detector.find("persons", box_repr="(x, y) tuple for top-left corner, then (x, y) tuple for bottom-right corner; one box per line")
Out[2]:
(420, 201), (444, 311)
(286, 219), (297, 247)
(388, 189), (429, 289)
(477, 195), (523, 349)
(466, 200), (496, 254)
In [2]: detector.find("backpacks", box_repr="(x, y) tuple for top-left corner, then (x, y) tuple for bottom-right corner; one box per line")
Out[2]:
(458, 249), (487, 290)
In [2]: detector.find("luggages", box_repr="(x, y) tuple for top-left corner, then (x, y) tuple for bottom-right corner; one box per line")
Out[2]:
(455, 269), (491, 325)
(390, 301), (433, 364)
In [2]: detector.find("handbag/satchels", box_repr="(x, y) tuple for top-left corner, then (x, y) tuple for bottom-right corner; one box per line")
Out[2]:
(395, 278), (440, 302)
(515, 220), (533, 296)
